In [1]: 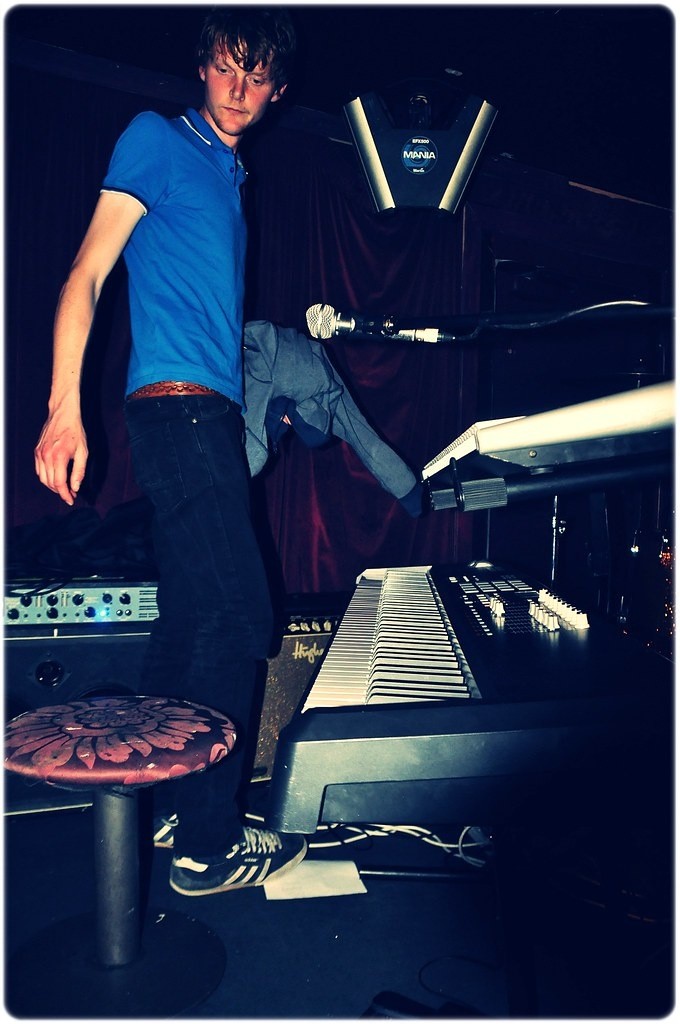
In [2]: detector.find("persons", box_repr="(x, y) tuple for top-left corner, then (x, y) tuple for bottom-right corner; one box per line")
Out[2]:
(35, 6), (308, 897)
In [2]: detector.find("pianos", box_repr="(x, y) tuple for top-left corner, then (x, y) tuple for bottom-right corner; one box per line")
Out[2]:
(269, 378), (676, 917)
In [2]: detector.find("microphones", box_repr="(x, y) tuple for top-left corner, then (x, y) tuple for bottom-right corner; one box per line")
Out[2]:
(305, 304), (457, 342)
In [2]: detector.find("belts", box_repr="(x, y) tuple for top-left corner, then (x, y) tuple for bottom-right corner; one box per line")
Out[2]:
(127, 382), (215, 398)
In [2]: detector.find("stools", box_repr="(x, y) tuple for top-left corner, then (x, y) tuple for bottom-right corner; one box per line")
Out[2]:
(6, 694), (240, 1017)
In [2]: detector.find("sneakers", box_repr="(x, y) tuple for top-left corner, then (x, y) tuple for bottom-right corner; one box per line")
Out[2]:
(169, 825), (307, 896)
(153, 813), (179, 848)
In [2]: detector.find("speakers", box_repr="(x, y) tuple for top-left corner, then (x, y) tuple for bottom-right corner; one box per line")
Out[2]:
(6, 580), (162, 816)
(248, 610), (344, 783)
